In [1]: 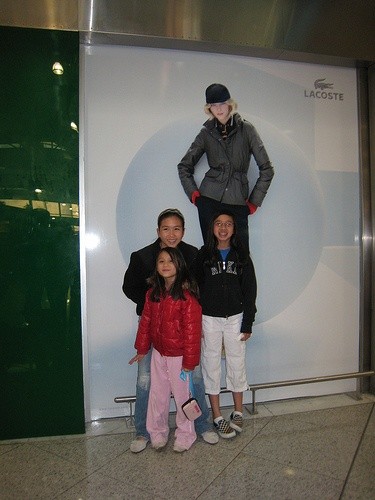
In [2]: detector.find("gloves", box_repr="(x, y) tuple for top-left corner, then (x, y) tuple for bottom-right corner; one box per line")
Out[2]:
(246, 199), (257, 214)
(192, 191), (201, 204)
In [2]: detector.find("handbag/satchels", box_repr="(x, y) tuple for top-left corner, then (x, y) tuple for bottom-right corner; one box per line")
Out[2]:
(182, 398), (202, 421)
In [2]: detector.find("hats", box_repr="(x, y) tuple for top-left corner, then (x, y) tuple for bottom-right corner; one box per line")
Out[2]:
(205, 84), (230, 103)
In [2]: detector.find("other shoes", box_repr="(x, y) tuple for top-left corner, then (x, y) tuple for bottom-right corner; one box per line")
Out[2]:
(201, 428), (219, 444)
(229, 412), (243, 432)
(151, 443), (167, 448)
(213, 418), (236, 438)
(173, 446), (185, 452)
(131, 435), (149, 452)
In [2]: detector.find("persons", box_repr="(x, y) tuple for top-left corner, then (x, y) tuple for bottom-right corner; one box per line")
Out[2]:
(178, 84), (274, 258)
(129, 247), (202, 453)
(194, 212), (257, 438)
(122, 209), (219, 452)
